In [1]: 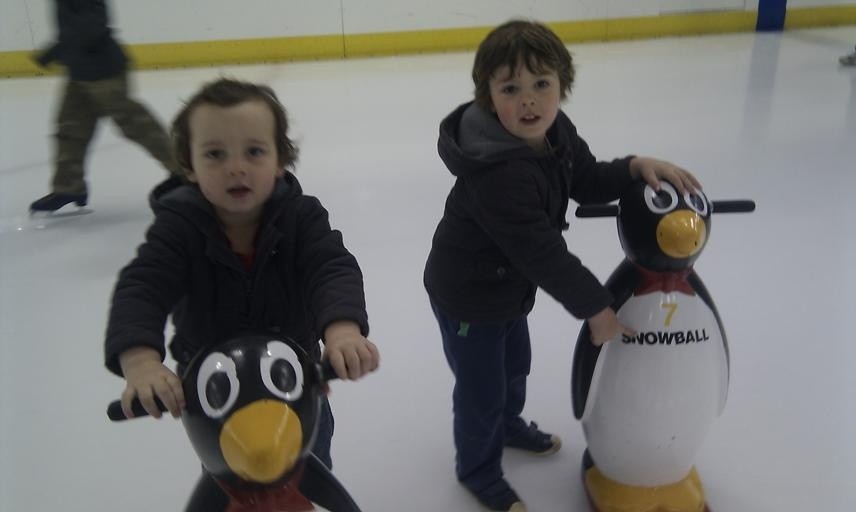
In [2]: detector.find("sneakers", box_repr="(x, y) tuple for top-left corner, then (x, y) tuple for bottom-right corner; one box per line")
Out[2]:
(457, 468), (528, 511)
(503, 415), (564, 457)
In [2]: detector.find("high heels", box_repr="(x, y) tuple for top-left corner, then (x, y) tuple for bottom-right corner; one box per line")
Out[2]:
(28, 188), (89, 212)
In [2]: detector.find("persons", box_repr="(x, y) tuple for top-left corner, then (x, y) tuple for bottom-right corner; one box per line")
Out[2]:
(101, 72), (381, 468)
(837, 44), (856, 68)
(28, 0), (172, 211)
(421, 18), (705, 510)
